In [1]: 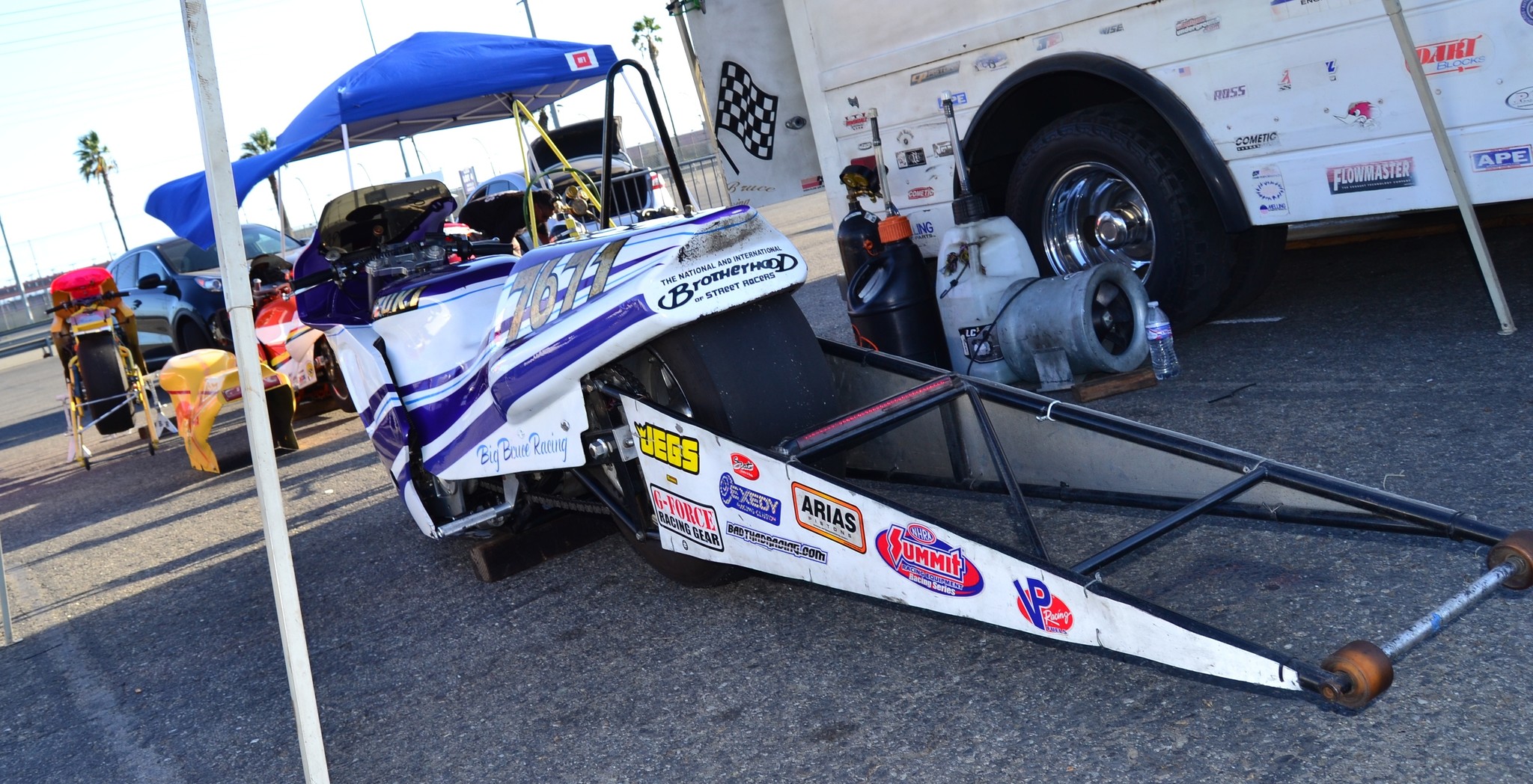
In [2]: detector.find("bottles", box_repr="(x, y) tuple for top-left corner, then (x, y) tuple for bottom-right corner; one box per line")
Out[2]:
(1144, 301), (1180, 381)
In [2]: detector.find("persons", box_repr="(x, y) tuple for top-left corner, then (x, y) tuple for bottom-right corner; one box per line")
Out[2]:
(458, 189), (560, 255)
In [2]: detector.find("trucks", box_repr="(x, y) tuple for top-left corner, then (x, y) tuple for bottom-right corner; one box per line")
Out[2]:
(681, 1), (1533, 339)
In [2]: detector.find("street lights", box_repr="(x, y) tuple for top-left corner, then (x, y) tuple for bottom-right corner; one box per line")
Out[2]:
(515, 0), (560, 129)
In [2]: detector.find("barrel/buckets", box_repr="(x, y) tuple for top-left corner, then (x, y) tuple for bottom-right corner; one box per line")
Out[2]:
(935, 192), (1040, 384)
(847, 215), (955, 382)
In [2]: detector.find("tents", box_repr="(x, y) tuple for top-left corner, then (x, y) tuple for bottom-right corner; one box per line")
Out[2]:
(137, 29), (700, 275)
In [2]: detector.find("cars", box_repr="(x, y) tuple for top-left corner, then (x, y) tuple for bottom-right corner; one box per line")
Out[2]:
(248, 180), (519, 415)
(456, 116), (676, 255)
(106, 223), (311, 371)
